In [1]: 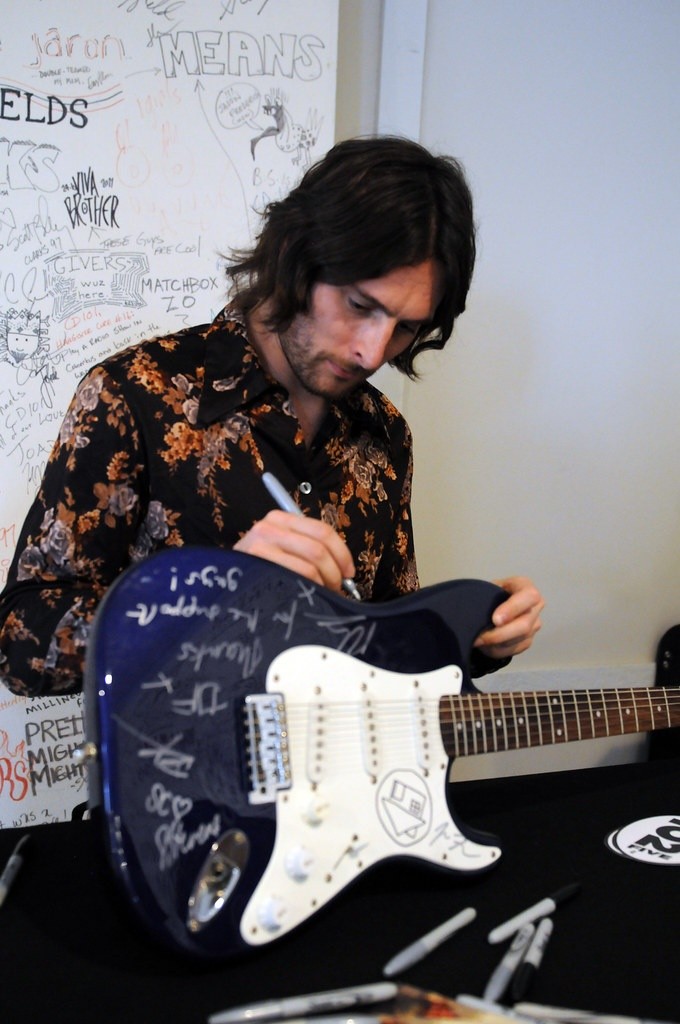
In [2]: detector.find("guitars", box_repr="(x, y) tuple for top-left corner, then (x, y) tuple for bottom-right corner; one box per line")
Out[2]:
(81, 542), (680, 964)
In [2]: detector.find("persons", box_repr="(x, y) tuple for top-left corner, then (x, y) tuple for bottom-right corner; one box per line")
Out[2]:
(1, 137), (547, 698)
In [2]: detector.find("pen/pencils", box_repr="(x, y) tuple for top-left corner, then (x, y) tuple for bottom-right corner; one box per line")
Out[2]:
(208, 982), (399, 1024)
(378, 908), (478, 977)
(261, 472), (363, 600)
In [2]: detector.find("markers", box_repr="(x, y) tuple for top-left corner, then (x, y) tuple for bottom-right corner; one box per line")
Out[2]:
(455, 878), (643, 1024)
